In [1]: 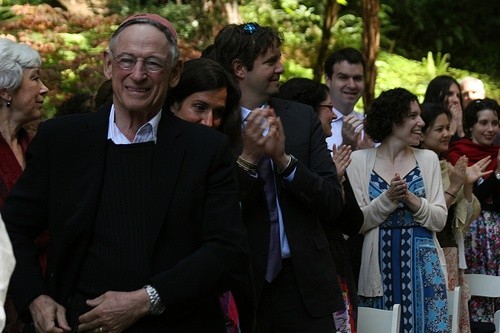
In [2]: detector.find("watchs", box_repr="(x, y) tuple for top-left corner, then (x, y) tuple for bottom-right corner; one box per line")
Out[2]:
(143, 283), (164, 314)
(276, 152), (298, 177)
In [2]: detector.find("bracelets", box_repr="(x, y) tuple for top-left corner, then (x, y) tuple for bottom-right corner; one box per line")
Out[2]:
(445, 189), (457, 197)
(235, 155), (258, 172)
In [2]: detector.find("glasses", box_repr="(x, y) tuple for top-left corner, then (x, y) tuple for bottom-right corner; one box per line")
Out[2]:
(316, 104), (333, 111)
(110, 49), (169, 73)
(244, 23), (257, 36)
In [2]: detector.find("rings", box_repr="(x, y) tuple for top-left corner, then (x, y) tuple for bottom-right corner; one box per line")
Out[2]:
(99, 326), (103, 333)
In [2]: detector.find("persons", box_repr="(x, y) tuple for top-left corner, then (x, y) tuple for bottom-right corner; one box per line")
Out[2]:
(0, 13), (500, 333)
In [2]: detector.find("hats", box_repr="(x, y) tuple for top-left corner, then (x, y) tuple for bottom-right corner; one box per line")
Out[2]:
(111, 13), (177, 46)
